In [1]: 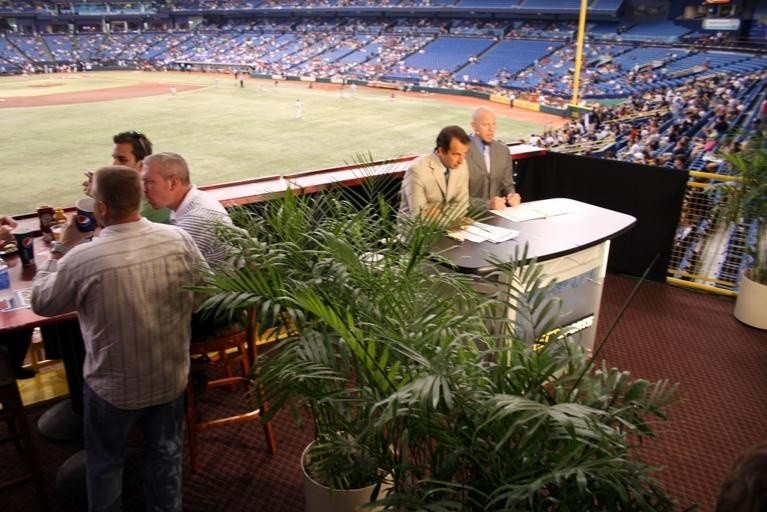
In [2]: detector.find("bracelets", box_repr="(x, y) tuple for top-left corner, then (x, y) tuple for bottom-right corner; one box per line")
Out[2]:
(50, 244), (68, 255)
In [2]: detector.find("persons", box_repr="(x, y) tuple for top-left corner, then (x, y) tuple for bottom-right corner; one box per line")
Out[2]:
(530, 19), (767, 276)
(464, 107), (522, 210)
(142, 151), (246, 346)
(38, 387), (91, 512)
(0, 0), (530, 100)
(0, 214), (35, 380)
(397, 125), (471, 235)
(82, 129), (171, 223)
(31, 166), (216, 511)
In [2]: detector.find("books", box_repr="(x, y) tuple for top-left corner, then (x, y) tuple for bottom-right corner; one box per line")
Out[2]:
(489, 202), (573, 222)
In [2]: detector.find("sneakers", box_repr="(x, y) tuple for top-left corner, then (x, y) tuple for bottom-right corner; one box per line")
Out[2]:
(0, 366), (36, 380)
(192, 355), (211, 395)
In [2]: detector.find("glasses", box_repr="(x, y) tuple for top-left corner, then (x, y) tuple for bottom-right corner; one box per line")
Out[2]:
(127, 130), (148, 156)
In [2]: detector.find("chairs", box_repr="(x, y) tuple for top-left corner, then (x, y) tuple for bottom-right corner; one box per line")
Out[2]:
(525, 76), (766, 293)
(0, 2), (766, 100)
(182, 305), (277, 472)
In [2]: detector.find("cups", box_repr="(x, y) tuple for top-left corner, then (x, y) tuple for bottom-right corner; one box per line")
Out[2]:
(75, 197), (97, 233)
(9, 226), (36, 268)
(50, 224), (65, 242)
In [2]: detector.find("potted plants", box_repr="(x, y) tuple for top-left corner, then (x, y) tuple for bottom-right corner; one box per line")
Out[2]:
(178, 155), (507, 512)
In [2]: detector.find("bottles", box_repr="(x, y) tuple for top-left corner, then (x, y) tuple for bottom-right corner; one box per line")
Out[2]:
(0, 257), (13, 300)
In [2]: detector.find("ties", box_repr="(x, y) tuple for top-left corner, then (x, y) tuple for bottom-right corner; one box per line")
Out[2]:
(444, 167), (450, 186)
(483, 145), (491, 175)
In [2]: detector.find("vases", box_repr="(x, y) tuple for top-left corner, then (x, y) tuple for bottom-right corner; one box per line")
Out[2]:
(708, 268), (766, 329)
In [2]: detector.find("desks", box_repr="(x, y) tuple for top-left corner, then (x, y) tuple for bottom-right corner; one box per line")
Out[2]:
(403, 197), (639, 394)
